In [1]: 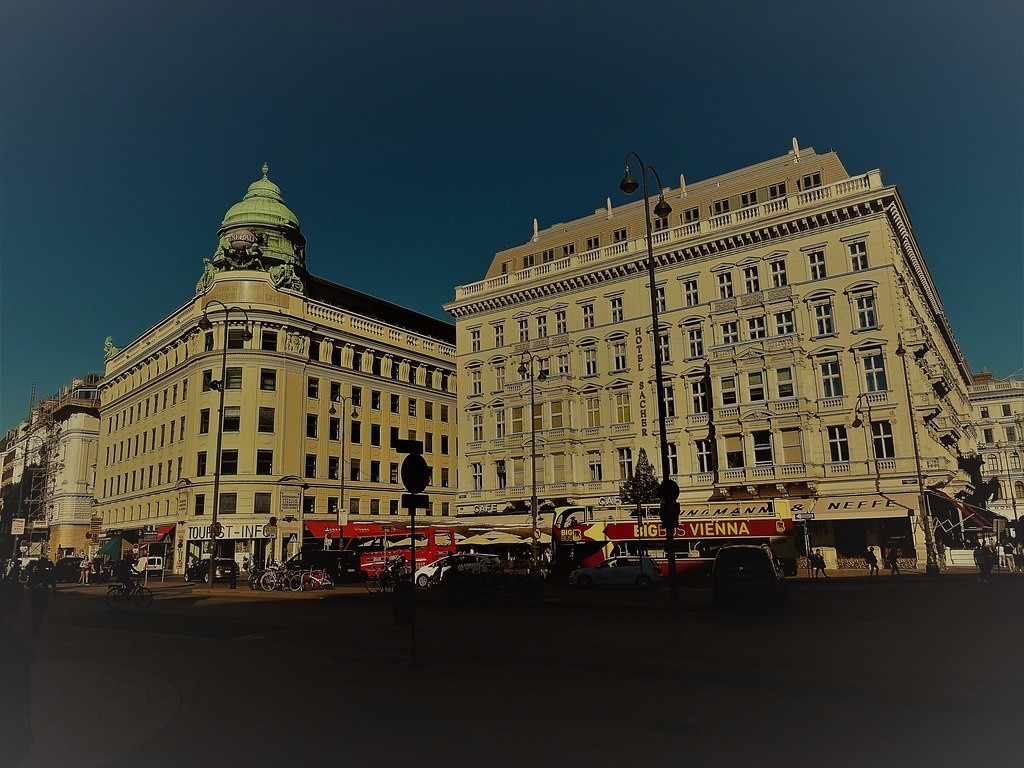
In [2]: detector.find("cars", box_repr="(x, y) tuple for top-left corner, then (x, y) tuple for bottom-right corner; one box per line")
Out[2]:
(184, 558), (241, 584)
(53, 555), (86, 583)
(31, 561), (54, 584)
(415, 553), (501, 591)
(568, 555), (664, 592)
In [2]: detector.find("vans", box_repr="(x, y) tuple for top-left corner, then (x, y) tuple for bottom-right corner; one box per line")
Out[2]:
(130, 555), (164, 577)
(282, 550), (357, 581)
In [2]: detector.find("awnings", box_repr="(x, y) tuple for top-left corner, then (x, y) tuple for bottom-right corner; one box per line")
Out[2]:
(930, 493), (1010, 529)
(679, 493), (921, 522)
(303, 521), (422, 538)
(139, 525), (175, 544)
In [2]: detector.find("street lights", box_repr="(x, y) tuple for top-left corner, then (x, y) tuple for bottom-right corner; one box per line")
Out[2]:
(619, 151), (674, 609)
(518, 349), (549, 573)
(894, 332), (941, 578)
(11, 436), (49, 581)
(195, 300), (253, 594)
(327, 392), (358, 584)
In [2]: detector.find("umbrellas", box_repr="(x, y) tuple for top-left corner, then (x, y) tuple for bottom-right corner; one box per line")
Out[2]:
(358, 532), (552, 562)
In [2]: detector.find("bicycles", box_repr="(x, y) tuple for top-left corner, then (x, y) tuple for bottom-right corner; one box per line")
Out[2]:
(245, 562), (326, 592)
(505, 564), (546, 600)
(364, 565), (413, 598)
(105, 571), (153, 610)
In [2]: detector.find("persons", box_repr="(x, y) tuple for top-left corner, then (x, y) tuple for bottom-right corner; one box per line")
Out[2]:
(391, 548), (553, 583)
(813, 549), (830, 579)
(868, 537), (1024, 579)
(8, 553), (141, 600)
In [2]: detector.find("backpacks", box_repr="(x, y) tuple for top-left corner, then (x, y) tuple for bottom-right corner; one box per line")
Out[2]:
(866, 554), (872, 564)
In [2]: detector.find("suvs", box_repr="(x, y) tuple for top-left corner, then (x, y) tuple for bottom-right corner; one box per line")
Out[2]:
(709, 541), (785, 612)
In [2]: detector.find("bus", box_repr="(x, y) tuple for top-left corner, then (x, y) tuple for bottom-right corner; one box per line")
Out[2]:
(552, 499), (798, 579)
(338, 525), (459, 579)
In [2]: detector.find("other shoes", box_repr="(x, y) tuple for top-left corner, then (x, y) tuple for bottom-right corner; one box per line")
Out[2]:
(825, 576), (831, 579)
(983, 579), (989, 583)
(977, 576), (981, 583)
